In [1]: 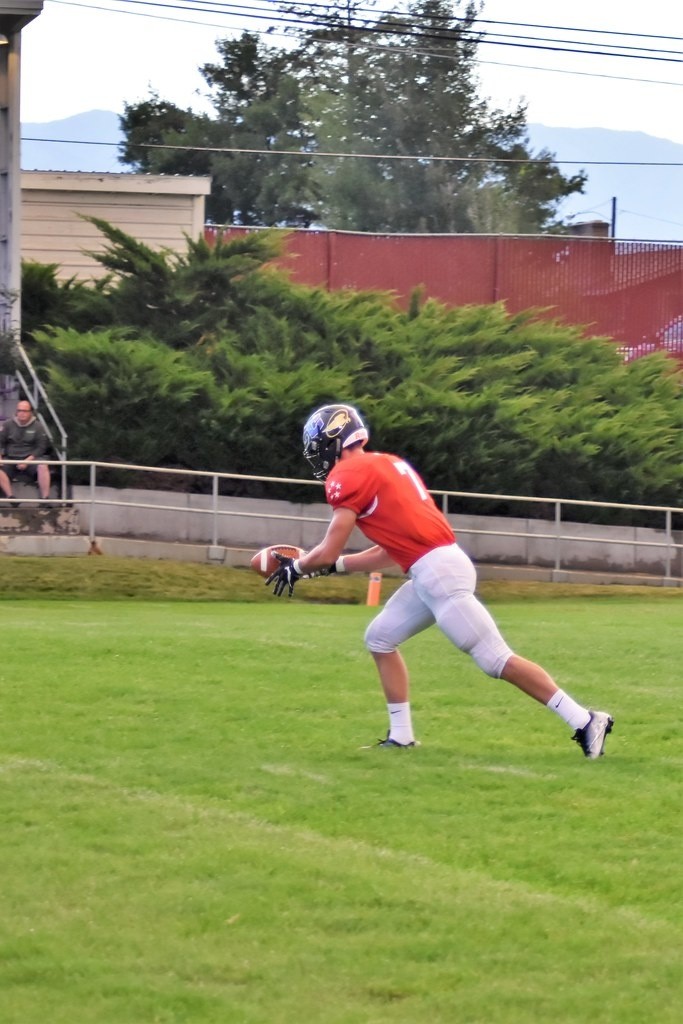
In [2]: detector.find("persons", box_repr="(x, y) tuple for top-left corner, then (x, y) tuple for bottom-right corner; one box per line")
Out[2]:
(0, 400), (61, 508)
(265, 404), (614, 760)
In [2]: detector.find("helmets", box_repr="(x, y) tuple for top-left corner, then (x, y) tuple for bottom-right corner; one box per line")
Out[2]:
(303, 403), (369, 480)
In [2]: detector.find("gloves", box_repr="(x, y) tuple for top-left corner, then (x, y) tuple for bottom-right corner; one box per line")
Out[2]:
(300, 556), (346, 579)
(263, 551), (303, 598)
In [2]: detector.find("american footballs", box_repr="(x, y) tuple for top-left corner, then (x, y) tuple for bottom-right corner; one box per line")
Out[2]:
(249, 543), (313, 579)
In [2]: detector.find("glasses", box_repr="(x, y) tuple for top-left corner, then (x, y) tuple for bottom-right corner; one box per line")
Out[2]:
(16, 409), (31, 413)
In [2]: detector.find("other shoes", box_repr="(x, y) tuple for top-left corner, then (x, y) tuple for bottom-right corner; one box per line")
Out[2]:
(9, 495), (20, 507)
(37, 503), (52, 508)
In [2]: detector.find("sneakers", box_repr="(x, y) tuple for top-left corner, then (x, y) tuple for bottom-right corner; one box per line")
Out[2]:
(372, 729), (416, 749)
(570, 709), (615, 758)
